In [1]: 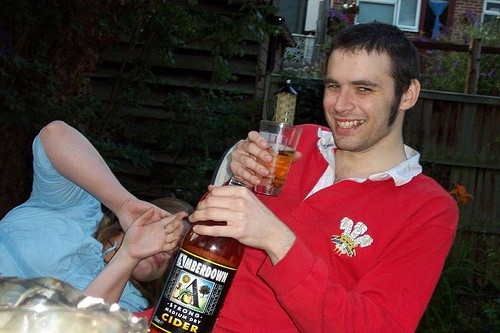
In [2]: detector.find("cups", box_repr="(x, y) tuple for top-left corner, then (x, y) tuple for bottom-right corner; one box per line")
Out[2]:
(254, 119), (303, 196)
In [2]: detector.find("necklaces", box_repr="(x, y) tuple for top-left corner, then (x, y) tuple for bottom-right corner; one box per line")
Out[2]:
(107, 239), (118, 253)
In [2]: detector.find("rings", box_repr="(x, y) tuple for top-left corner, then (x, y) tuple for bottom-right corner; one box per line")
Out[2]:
(164, 228), (168, 234)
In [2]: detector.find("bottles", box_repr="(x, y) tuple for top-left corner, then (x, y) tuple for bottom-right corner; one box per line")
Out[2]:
(147, 176), (252, 333)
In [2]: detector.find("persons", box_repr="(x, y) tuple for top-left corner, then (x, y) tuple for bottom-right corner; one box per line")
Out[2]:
(133, 23), (459, 333)
(0, 121), (197, 314)
